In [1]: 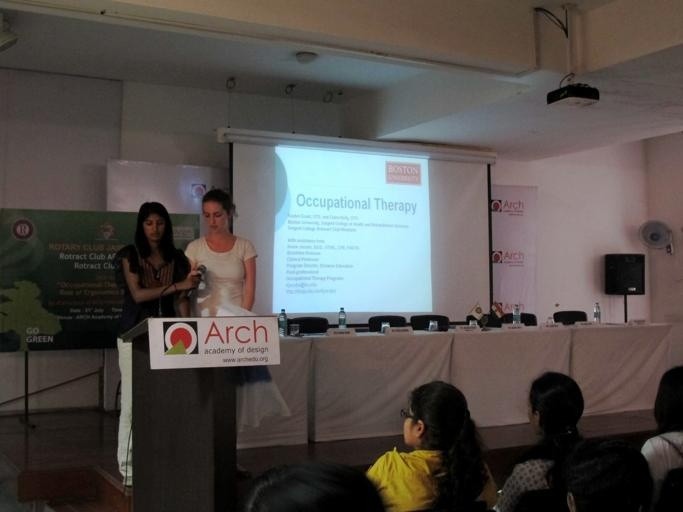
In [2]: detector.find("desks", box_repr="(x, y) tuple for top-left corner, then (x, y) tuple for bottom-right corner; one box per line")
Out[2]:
(235, 320), (683, 450)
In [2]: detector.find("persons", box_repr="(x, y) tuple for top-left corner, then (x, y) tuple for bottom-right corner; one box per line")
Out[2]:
(112, 202), (202, 488)
(183, 189), (293, 480)
(640, 365), (683, 512)
(364, 381), (498, 512)
(552, 440), (654, 512)
(230, 462), (386, 512)
(493, 372), (586, 512)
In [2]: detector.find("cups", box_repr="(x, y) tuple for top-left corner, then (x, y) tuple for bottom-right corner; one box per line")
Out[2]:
(429, 320), (438, 331)
(381, 322), (390, 333)
(289, 324), (300, 336)
(469, 320), (478, 327)
(546, 319), (555, 328)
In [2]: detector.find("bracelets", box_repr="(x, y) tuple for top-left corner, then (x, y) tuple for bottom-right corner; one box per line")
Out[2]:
(173, 282), (178, 292)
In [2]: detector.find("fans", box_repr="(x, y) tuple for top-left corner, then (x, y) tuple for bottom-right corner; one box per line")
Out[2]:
(639, 220), (675, 255)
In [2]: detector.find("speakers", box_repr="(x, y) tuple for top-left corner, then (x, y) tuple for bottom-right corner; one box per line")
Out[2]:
(605, 254), (645, 294)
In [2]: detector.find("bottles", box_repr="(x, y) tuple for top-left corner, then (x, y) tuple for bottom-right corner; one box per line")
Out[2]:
(338, 307), (346, 329)
(278, 308), (288, 337)
(594, 302), (600, 325)
(513, 304), (520, 329)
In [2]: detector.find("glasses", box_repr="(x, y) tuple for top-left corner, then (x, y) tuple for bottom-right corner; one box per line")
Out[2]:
(401, 408), (417, 421)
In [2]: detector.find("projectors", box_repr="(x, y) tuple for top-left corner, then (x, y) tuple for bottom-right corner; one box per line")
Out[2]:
(547, 84), (599, 107)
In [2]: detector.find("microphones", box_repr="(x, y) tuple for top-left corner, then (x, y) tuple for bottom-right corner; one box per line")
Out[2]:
(187, 265), (205, 297)
